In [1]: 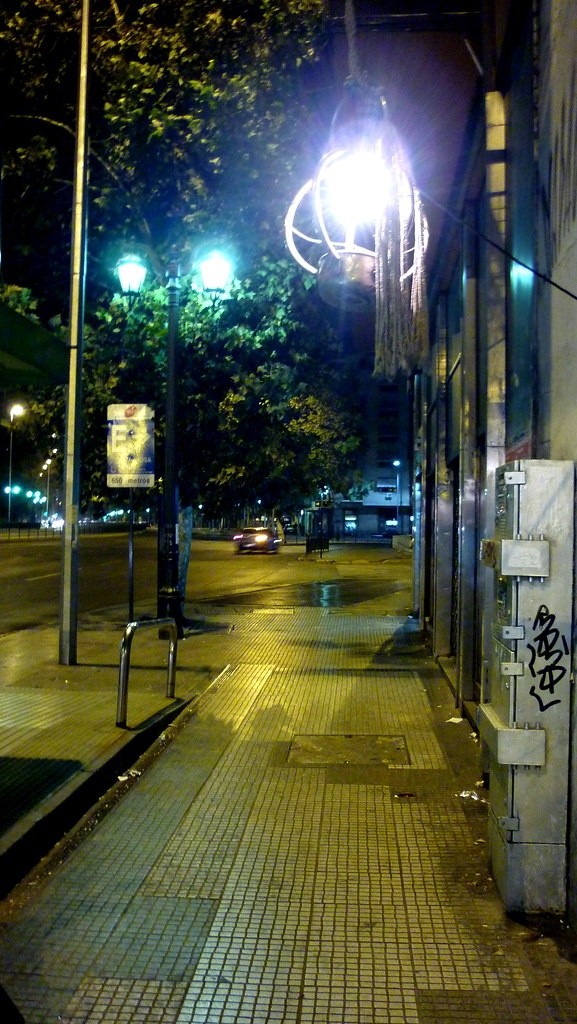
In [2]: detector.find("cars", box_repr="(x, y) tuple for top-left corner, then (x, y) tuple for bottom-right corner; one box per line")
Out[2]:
(233, 527), (280, 553)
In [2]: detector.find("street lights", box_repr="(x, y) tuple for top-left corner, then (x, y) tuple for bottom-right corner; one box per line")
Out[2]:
(393, 460), (401, 533)
(8, 404), (24, 540)
(113, 241), (238, 640)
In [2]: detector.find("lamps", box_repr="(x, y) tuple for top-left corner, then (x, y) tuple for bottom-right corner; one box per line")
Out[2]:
(285, 0), (429, 379)
